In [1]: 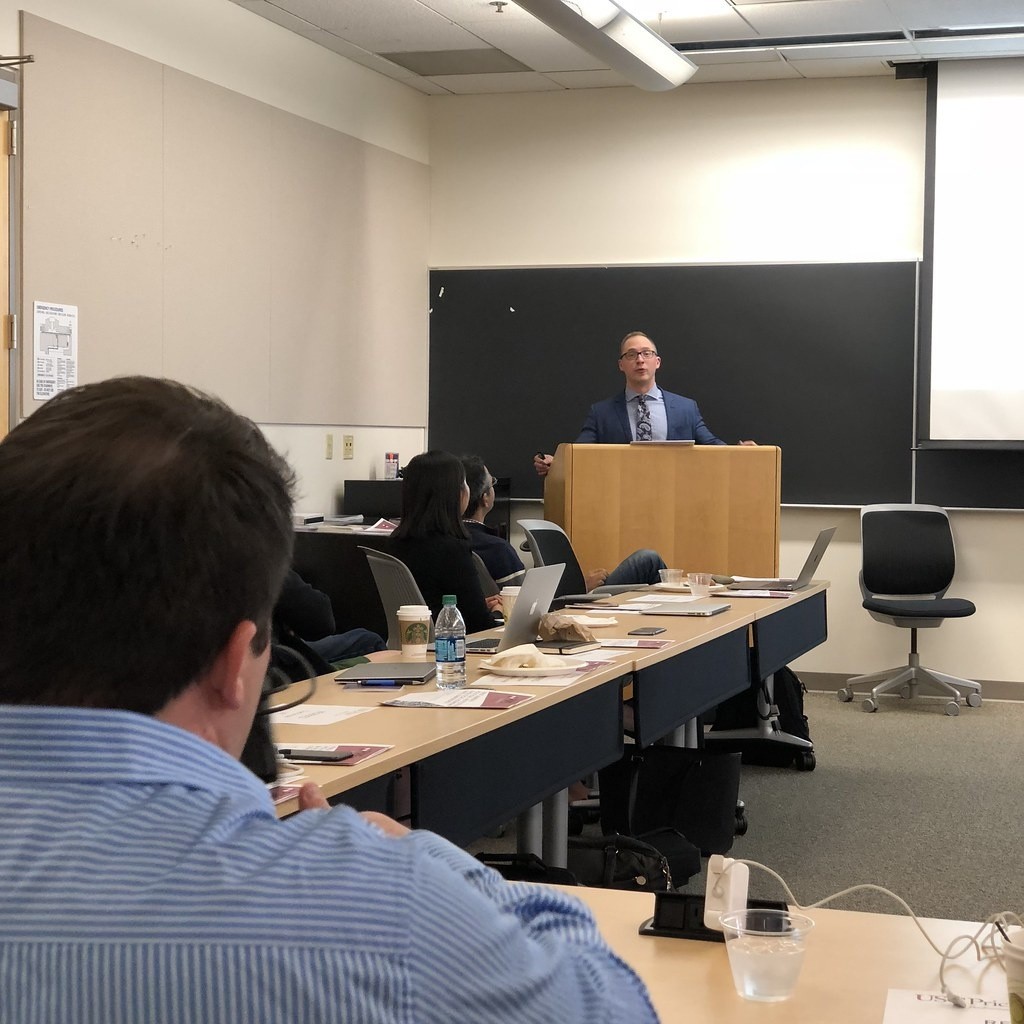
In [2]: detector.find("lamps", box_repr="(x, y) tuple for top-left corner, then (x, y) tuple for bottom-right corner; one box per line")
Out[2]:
(511, 0), (696, 94)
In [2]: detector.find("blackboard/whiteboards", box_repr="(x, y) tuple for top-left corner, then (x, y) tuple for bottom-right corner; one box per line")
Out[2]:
(427, 259), (1023, 515)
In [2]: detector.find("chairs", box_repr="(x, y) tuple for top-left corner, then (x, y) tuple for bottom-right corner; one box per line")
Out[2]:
(357, 543), (437, 649)
(838, 504), (982, 716)
(515, 518), (648, 603)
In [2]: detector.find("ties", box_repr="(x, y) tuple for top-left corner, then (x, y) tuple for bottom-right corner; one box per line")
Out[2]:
(636, 395), (653, 441)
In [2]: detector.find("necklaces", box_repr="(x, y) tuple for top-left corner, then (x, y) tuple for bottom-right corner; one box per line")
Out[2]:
(463, 518), (483, 525)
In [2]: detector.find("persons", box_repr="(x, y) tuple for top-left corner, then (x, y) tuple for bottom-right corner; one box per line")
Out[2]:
(386, 450), (668, 819)
(534, 332), (757, 475)
(269, 568), (388, 672)
(0, 375), (660, 1024)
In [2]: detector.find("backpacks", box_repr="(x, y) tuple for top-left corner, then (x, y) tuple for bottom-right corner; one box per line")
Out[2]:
(708, 665), (808, 766)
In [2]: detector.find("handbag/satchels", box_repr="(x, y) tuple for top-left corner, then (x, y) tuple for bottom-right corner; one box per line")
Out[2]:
(599, 744), (742, 857)
(569, 833), (681, 892)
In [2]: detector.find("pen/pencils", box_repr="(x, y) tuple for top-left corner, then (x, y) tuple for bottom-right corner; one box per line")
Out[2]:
(358, 680), (423, 685)
(537, 452), (551, 467)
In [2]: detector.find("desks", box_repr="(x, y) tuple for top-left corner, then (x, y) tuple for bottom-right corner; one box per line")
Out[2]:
(344, 478), (409, 524)
(272, 578), (1024, 1024)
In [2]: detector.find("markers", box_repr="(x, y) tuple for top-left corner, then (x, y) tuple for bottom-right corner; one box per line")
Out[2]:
(386, 453), (390, 462)
(394, 454), (398, 463)
(389, 453), (393, 463)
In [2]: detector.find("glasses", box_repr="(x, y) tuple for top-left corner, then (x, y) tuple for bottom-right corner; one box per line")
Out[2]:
(480, 477), (497, 498)
(619, 350), (658, 360)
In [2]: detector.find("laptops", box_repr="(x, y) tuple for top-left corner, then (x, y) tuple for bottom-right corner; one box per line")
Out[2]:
(428, 562), (566, 652)
(728, 526), (838, 589)
(335, 663), (437, 683)
(639, 602), (731, 616)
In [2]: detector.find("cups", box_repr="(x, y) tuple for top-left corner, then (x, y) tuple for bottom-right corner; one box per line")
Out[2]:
(658, 568), (684, 583)
(500, 586), (521, 629)
(686, 573), (713, 595)
(1000, 927), (1024, 1024)
(395, 605), (432, 658)
(717, 909), (816, 1002)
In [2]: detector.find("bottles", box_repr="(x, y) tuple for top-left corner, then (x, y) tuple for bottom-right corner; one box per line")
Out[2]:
(435, 595), (467, 690)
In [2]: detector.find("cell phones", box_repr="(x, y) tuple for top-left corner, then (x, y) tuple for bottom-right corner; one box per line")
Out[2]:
(278, 749), (353, 759)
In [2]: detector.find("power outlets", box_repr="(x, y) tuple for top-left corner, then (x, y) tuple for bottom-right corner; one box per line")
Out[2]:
(342, 435), (353, 458)
(326, 433), (334, 460)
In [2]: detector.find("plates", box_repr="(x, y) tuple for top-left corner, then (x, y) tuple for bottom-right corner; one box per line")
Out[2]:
(478, 657), (590, 678)
(580, 617), (618, 628)
(654, 582), (725, 593)
